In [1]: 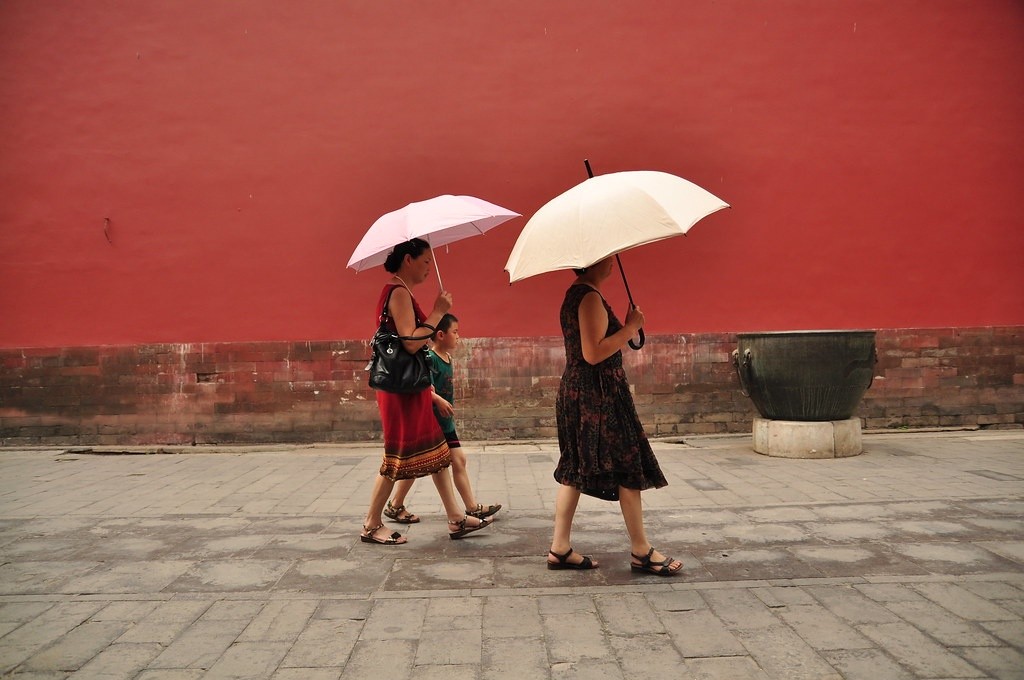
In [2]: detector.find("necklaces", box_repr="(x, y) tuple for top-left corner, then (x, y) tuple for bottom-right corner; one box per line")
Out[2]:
(394, 275), (415, 299)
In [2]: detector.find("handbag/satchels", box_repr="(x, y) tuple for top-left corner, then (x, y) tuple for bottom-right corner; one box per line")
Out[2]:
(365, 285), (436, 394)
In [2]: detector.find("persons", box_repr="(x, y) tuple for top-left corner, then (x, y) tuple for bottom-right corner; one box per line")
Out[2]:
(546, 256), (684, 578)
(358, 237), (495, 547)
(383, 312), (503, 525)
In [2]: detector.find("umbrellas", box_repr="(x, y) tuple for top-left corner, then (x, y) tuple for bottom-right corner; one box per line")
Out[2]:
(346, 194), (524, 292)
(503, 158), (731, 350)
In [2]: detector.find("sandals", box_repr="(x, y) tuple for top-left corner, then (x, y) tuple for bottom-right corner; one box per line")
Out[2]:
(465, 504), (502, 518)
(448, 514), (494, 540)
(631, 547), (684, 576)
(361, 523), (407, 545)
(384, 500), (420, 523)
(547, 548), (600, 570)
